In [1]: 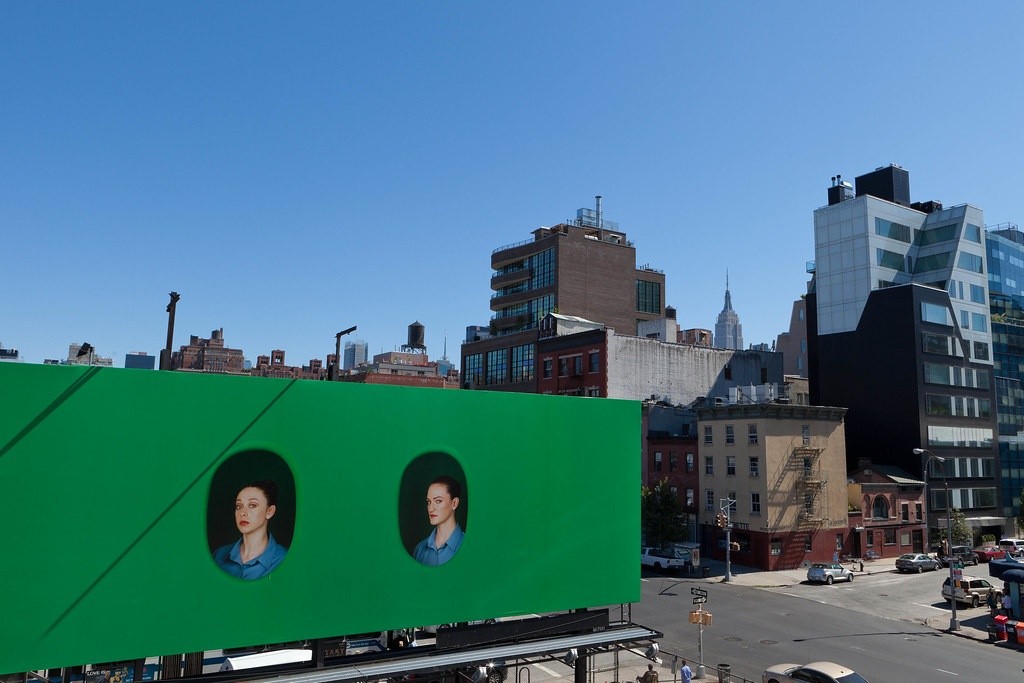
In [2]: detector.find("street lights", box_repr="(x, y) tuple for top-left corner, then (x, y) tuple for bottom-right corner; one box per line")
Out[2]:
(913, 448), (962, 629)
(922, 455), (946, 551)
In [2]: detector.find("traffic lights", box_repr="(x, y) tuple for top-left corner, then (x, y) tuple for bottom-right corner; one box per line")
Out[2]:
(733, 544), (740, 551)
(716, 513), (722, 528)
(722, 515), (728, 528)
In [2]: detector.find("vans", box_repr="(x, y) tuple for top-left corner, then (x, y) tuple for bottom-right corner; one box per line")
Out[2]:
(1000, 539), (1024, 556)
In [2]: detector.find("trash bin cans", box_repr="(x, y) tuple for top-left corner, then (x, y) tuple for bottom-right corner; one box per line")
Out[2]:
(987, 624), (999, 641)
(717, 664), (731, 683)
(995, 615), (1007, 640)
(1015, 622), (1024, 644)
(1005, 620), (1018, 643)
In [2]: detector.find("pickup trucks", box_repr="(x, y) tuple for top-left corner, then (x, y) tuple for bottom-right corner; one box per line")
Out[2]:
(641, 547), (685, 571)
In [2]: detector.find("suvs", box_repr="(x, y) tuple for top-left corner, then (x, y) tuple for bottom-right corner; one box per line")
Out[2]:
(943, 546), (979, 567)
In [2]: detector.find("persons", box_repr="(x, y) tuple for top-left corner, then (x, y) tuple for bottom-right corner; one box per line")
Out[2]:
(98, 667), (128, 683)
(986, 589), (1014, 618)
(681, 660), (692, 683)
(413, 476), (464, 567)
(212, 480), (289, 581)
(636, 664), (658, 683)
(834, 549), (839, 563)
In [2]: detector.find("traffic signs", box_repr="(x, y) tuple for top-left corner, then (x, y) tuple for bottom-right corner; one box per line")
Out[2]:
(691, 588), (707, 597)
(693, 597), (707, 604)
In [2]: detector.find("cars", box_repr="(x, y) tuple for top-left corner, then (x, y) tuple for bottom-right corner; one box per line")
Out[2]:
(895, 554), (940, 573)
(971, 546), (1006, 560)
(807, 560), (854, 585)
(762, 661), (870, 683)
(942, 575), (1002, 608)
